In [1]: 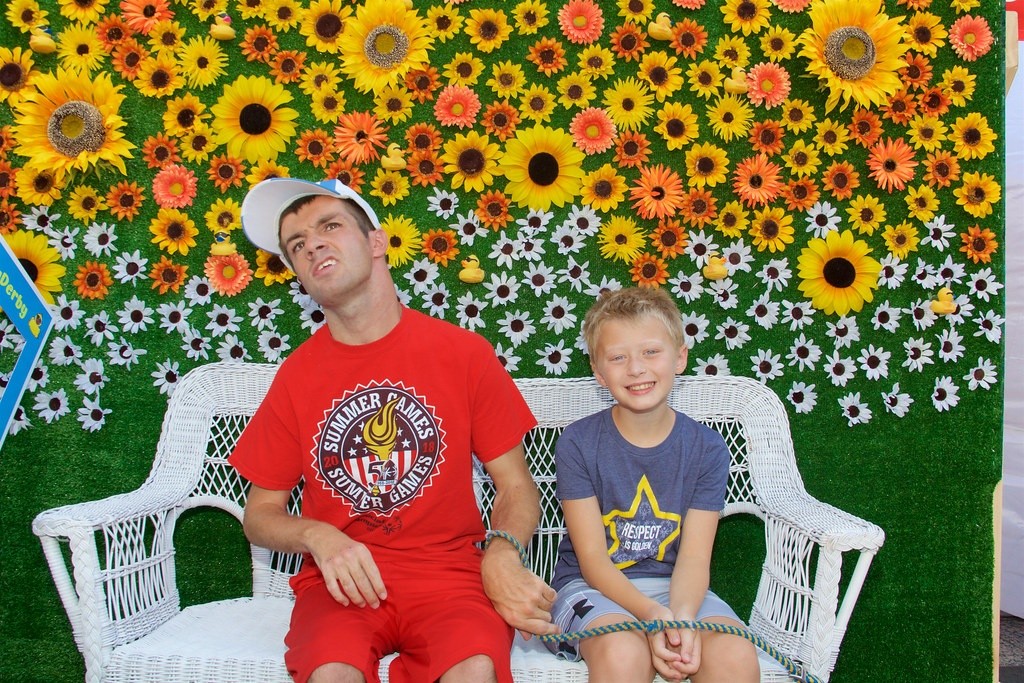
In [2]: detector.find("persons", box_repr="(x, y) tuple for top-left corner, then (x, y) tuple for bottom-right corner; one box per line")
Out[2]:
(227, 178), (563, 683)
(540, 285), (762, 683)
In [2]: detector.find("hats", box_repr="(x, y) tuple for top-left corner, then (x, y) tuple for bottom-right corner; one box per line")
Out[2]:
(241, 177), (381, 270)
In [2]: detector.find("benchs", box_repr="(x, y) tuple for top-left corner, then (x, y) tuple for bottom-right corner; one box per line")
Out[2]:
(33, 362), (886, 683)
